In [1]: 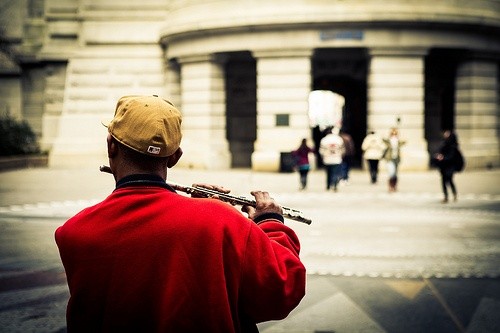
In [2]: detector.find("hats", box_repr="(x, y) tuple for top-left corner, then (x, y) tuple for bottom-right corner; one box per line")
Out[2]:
(101, 93), (183, 156)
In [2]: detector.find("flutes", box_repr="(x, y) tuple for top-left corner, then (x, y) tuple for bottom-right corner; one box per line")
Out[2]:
(99, 164), (311, 225)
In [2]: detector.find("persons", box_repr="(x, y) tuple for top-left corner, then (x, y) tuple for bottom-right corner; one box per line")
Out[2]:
(361, 129), (387, 183)
(382, 127), (406, 192)
(55, 94), (306, 333)
(318, 126), (346, 192)
(313, 125), (356, 181)
(289, 139), (318, 189)
(433, 127), (459, 203)
